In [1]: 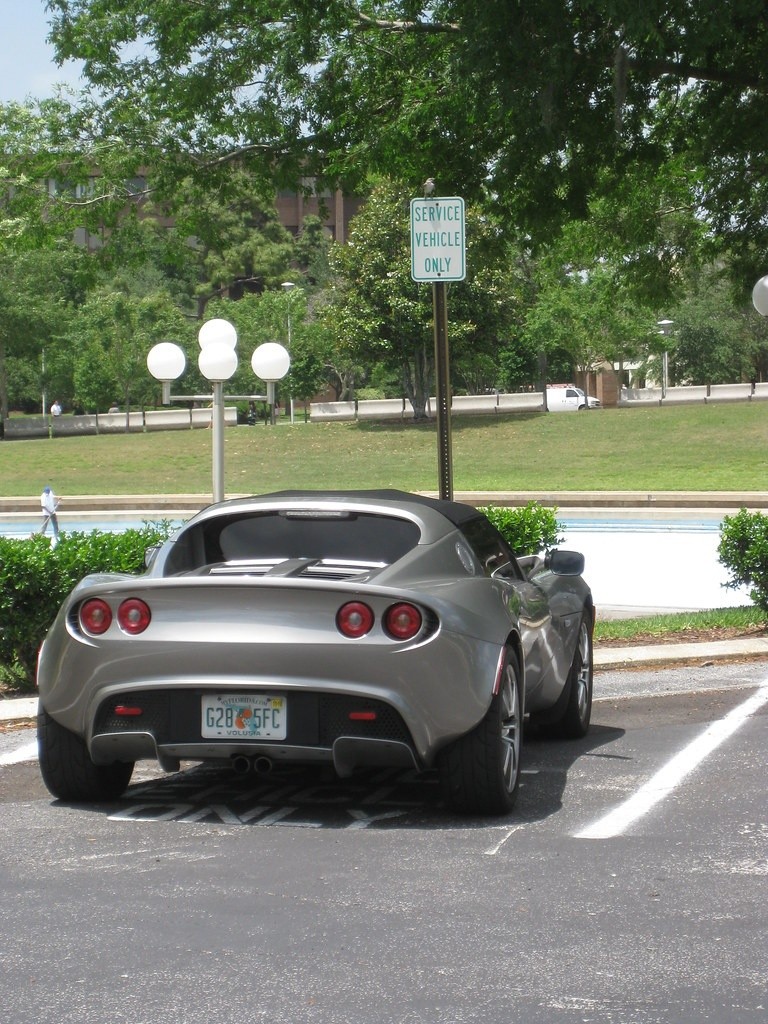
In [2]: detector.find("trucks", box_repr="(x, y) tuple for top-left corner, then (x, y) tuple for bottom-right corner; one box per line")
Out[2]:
(546, 384), (603, 423)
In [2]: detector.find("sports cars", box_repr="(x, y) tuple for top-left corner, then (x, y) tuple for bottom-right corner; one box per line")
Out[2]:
(37, 489), (596, 817)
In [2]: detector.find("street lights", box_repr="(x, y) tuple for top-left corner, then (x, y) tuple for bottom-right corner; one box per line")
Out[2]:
(147, 319), (290, 505)
(282, 282), (296, 425)
(658, 319), (673, 387)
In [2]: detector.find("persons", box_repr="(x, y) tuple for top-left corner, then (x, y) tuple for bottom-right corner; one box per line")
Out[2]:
(275, 400), (280, 423)
(41, 487), (59, 538)
(248, 400), (255, 425)
(51, 400), (120, 425)
(206, 385), (214, 430)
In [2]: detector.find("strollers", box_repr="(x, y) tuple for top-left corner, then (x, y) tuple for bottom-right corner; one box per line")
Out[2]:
(246, 411), (257, 425)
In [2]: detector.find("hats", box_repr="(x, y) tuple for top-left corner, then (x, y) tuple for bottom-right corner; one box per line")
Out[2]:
(43, 487), (50, 494)
